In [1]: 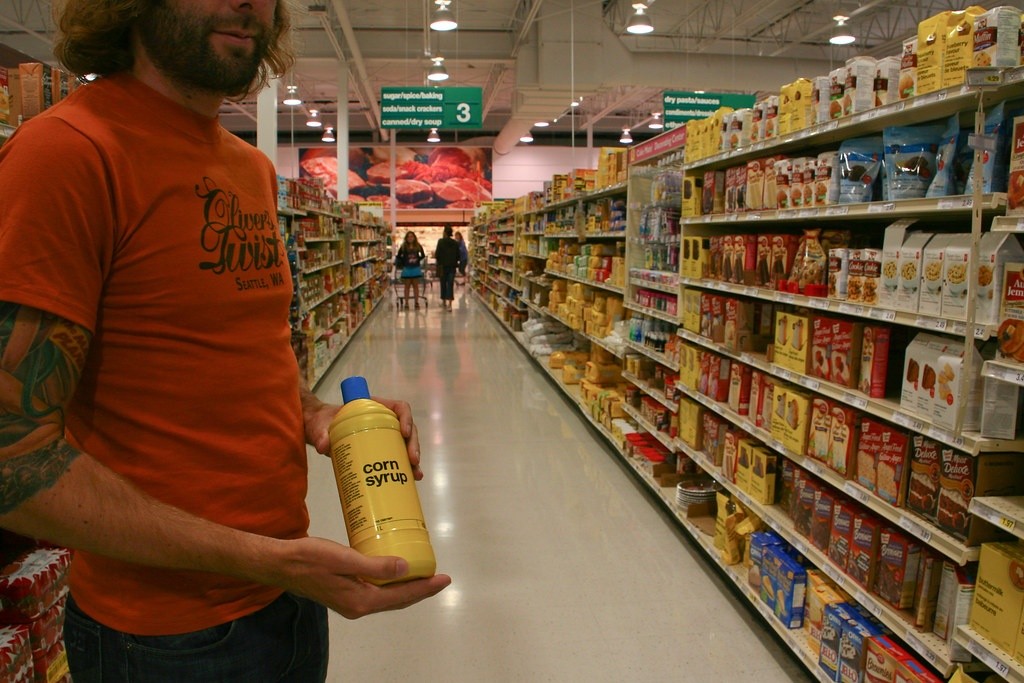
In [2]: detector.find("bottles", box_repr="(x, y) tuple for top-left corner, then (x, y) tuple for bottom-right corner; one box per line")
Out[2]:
(328, 376), (436, 587)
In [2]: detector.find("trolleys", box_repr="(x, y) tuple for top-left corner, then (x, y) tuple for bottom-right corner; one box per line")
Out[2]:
(393, 253), (429, 307)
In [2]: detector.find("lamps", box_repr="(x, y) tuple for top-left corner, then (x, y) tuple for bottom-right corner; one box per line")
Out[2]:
(430, 1), (458, 31)
(626, 0), (654, 34)
(829, 10), (855, 45)
(426, 31), (449, 80)
(283, 82), (335, 143)
(427, 128), (441, 143)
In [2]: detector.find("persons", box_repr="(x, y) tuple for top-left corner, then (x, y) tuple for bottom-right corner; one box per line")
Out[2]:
(0, 0), (452, 683)
(435, 227), (459, 310)
(455, 232), (468, 284)
(397, 231), (425, 308)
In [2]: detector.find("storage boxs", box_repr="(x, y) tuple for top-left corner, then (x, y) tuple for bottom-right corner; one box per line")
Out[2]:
(682, 159), (1023, 683)
(0, 63), (76, 126)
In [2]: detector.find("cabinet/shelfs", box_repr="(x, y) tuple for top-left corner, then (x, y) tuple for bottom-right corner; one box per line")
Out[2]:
(269, 176), (395, 392)
(673, 64), (1024, 683)
(465, 122), (680, 512)
